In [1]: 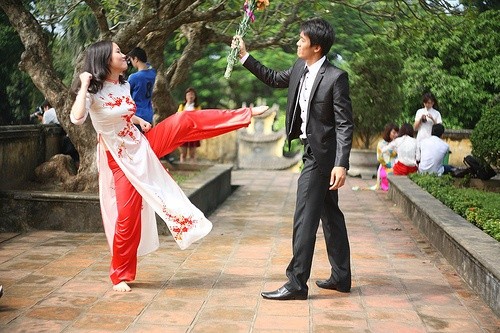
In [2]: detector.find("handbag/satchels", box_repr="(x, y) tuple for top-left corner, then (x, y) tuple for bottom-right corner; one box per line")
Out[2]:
(450, 167), (470, 178)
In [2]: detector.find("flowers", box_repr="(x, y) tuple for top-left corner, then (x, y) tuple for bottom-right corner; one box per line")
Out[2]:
(224, 0), (270, 79)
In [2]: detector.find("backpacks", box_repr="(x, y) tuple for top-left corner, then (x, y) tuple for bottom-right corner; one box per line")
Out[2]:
(463, 154), (497, 180)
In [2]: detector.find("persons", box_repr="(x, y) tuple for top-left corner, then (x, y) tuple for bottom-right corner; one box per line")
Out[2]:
(376, 124), (399, 191)
(230, 18), (354, 300)
(417, 123), (455, 176)
(127, 47), (157, 135)
(37, 100), (60, 125)
(413, 93), (443, 141)
(70, 41), (269, 292)
(177, 88), (202, 162)
(386, 124), (416, 176)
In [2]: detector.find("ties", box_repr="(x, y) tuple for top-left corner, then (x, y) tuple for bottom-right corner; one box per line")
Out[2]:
(288, 66), (309, 135)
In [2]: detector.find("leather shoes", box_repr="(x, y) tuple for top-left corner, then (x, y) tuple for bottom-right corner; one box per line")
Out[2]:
(261, 285), (308, 300)
(316, 280), (351, 292)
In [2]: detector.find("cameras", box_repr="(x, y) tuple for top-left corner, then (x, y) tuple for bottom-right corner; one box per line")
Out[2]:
(126, 59), (131, 66)
(30, 106), (44, 120)
